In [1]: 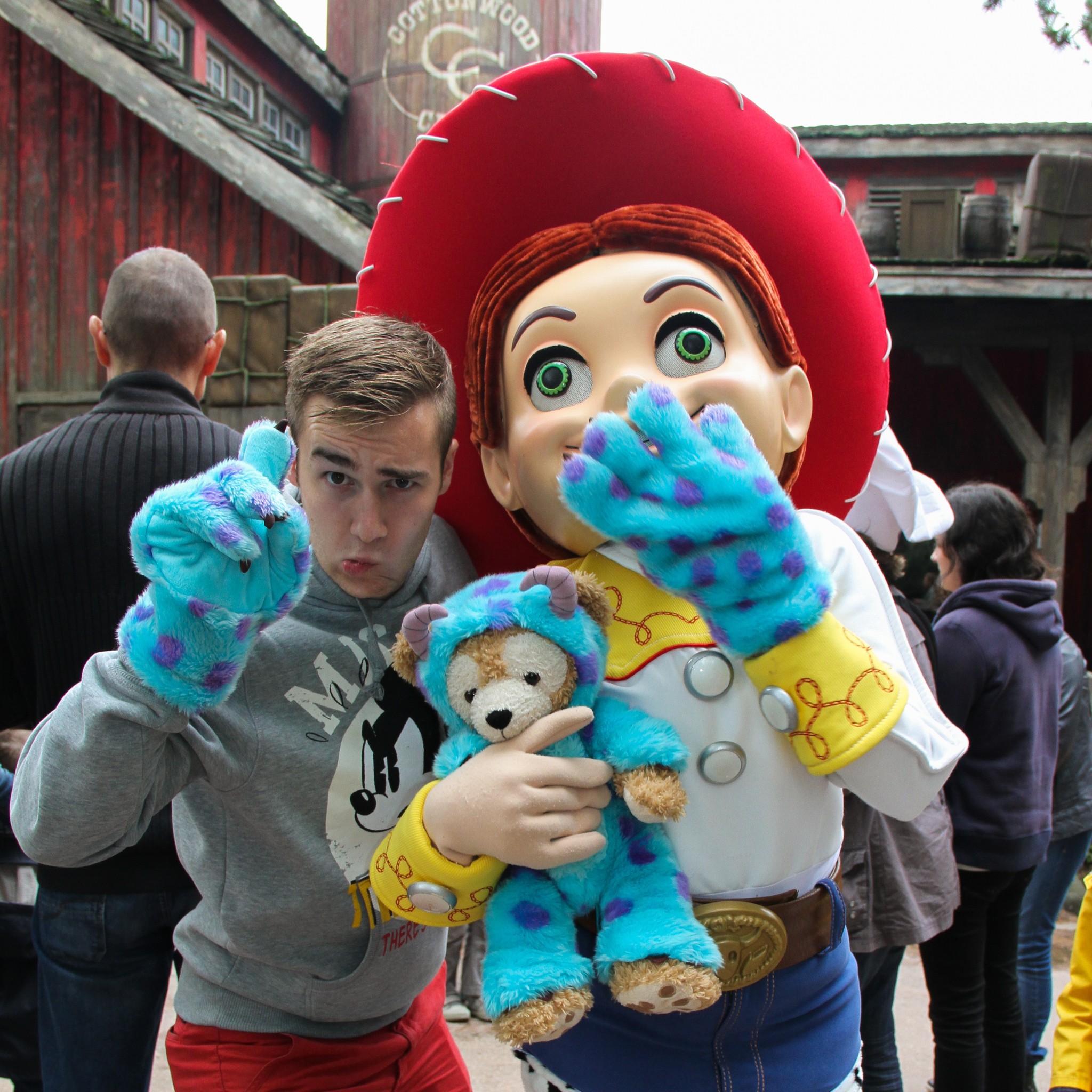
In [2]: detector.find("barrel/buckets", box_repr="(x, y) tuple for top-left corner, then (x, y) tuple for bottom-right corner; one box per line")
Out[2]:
(859, 207), (897, 258)
(960, 196), (1012, 260)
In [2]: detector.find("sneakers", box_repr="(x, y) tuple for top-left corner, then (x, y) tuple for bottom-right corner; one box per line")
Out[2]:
(463, 995), (492, 1022)
(442, 991), (471, 1020)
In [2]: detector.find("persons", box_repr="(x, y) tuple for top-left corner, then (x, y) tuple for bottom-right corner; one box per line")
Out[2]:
(843, 482), (1092, 1092)
(8, 313), (477, 1092)
(0, 246), (245, 1092)
(352, 47), (969, 1092)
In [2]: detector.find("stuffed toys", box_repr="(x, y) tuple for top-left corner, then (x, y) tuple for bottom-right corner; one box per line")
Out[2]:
(392, 566), (727, 1047)
